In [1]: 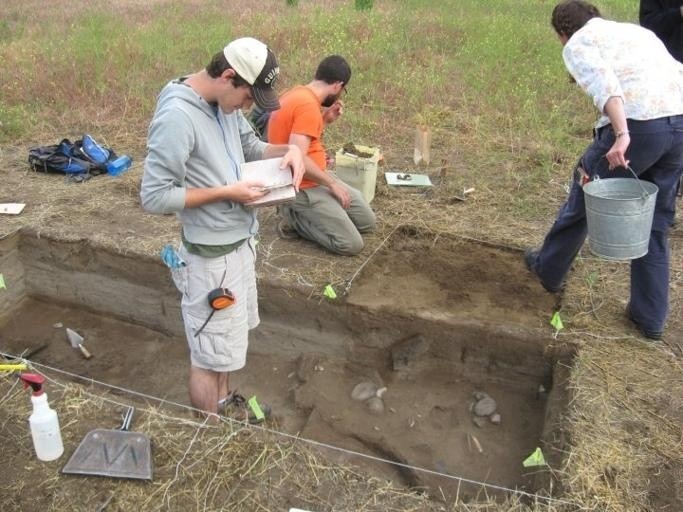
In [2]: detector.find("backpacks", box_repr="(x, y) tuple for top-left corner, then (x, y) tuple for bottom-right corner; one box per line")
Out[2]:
(29, 138), (117, 175)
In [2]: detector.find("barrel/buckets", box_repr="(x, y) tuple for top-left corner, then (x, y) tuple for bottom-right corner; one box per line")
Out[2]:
(581, 167), (659, 260)
(335, 145), (381, 206)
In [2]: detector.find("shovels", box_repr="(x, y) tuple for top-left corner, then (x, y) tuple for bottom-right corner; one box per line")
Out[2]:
(65, 327), (92, 358)
(453, 186), (476, 202)
(62, 406), (153, 483)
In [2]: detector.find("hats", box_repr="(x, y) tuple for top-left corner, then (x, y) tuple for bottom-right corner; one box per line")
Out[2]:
(224, 36), (281, 112)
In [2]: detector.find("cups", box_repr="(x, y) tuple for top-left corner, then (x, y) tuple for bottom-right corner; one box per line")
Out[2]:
(413, 124), (430, 169)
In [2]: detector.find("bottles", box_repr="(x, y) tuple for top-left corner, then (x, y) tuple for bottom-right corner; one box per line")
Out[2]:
(166, 245), (178, 268)
(107, 155), (134, 176)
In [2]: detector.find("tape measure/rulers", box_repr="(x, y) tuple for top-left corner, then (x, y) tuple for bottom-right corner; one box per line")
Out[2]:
(194, 289), (236, 338)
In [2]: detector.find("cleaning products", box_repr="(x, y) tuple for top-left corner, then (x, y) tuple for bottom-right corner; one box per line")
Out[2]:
(14, 372), (65, 462)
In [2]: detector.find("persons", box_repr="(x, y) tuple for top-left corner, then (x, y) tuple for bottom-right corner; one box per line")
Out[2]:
(264, 53), (374, 255)
(138, 37), (306, 425)
(524, 0), (683, 340)
(639, 0), (683, 226)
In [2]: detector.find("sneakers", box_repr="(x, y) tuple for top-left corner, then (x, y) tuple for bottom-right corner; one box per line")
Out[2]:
(276, 204), (299, 241)
(624, 301), (664, 340)
(526, 246), (563, 293)
(219, 388), (270, 424)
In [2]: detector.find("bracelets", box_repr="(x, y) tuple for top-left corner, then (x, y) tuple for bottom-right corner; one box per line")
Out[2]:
(613, 128), (629, 137)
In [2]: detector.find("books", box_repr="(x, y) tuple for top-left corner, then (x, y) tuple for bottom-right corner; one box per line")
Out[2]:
(240, 156), (296, 207)
